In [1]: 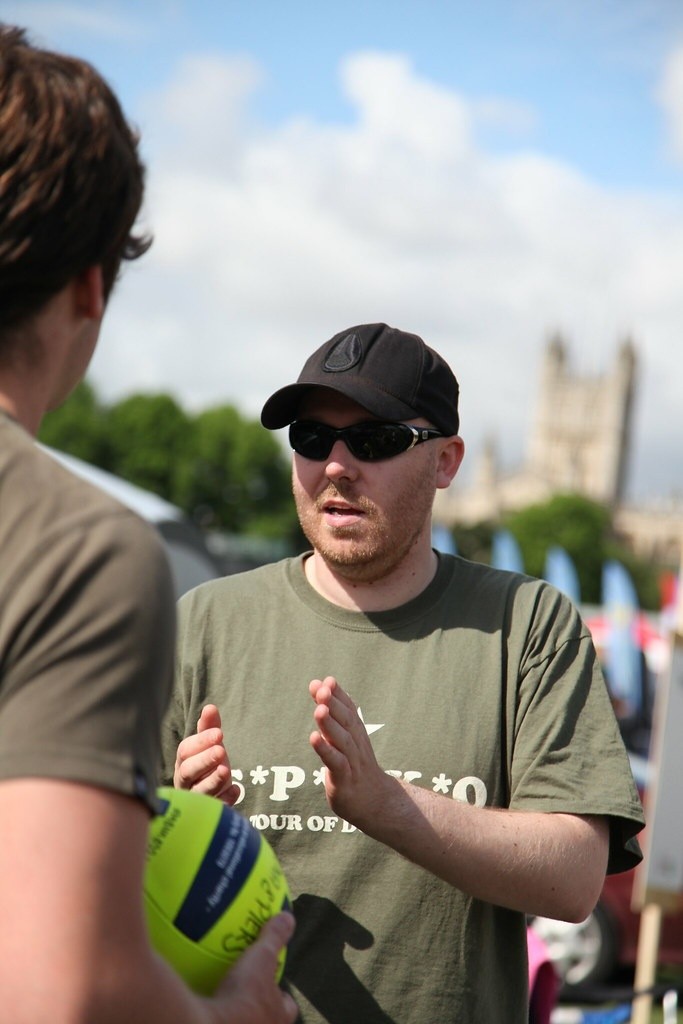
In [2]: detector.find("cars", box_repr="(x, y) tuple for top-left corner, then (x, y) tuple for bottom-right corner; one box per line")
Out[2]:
(524, 618), (682, 1005)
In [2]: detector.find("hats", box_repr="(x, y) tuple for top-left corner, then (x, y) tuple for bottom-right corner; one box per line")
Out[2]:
(261, 322), (461, 436)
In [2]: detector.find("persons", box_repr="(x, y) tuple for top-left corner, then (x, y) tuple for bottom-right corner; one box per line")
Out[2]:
(0, 26), (297, 1024)
(157, 322), (649, 1023)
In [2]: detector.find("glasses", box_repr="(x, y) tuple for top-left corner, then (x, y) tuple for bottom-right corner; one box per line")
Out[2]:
(289, 418), (442, 462)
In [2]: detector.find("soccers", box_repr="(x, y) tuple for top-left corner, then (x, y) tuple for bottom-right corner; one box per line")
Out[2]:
(130, 783), (292, 1001)
